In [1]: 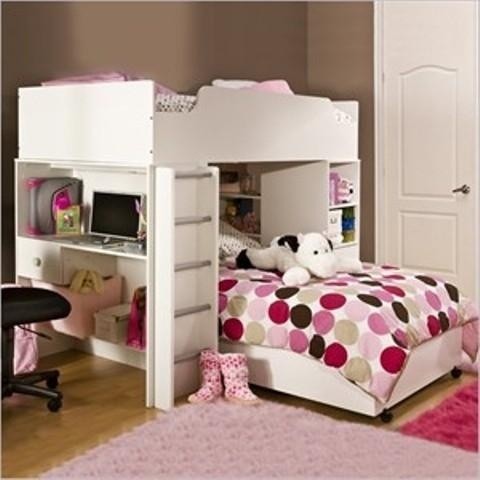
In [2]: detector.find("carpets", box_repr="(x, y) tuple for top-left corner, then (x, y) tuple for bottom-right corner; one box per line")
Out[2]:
(396, 380), (480, 453)
(34, 392), (480, 480)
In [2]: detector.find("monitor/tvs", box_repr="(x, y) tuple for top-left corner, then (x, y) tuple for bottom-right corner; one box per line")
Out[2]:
(89, 190), (145, 242)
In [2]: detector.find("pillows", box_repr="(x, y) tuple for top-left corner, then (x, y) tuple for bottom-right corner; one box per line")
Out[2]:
(218, 221), (262, 260)
(40, 70), (178, 95)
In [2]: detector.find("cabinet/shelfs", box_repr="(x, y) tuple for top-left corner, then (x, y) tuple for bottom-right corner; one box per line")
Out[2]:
(14, 158), (154, 408)
(219, 192), (261, 239)
(261, 161), (361, 261)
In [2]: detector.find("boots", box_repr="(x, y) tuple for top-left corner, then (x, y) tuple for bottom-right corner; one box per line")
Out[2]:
(188, 351), (223, 404)
(219, 353), (261, 406)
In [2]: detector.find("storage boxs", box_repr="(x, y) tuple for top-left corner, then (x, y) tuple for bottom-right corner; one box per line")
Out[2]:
(31, 273), (122, 340)
(94, 303), (130, 344)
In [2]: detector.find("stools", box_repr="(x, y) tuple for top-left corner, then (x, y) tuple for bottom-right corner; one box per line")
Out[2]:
(1, 287), (72, 412)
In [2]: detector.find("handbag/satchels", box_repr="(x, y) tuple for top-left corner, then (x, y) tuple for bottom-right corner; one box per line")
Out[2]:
(127, 287), (146, 347)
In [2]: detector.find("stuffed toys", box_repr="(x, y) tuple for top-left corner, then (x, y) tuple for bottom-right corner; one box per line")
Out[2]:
(337, 176), (353, 204)
(235, 230), (363, 287)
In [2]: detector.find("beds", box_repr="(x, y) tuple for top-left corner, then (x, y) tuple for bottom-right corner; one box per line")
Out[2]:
(18, 80), (359, 166)
(219, 264), (480, 422)
